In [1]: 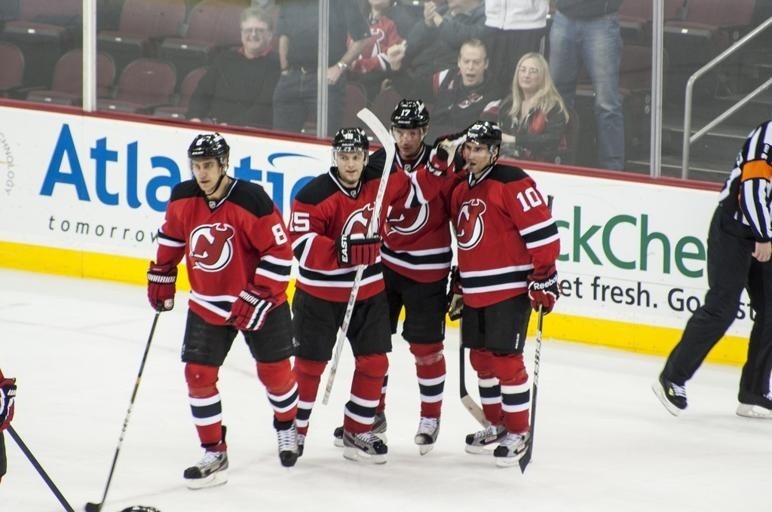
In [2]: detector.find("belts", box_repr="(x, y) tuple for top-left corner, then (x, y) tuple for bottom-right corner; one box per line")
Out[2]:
(293, 65), (317, 74)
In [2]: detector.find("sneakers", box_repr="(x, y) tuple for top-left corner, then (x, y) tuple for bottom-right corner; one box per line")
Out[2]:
(414, 416), (440, 444)
(494, 429), (530, 456)
(297, 431), (305, 458)
(333, 412), (387, 438)
(659, 371), (688, 410)
(273, 419), (297, 467)
(342, 426), (388, 454)
(464, 423), (506, 446)
(738, 390), (772, 410)
(184, 450), (229, 479)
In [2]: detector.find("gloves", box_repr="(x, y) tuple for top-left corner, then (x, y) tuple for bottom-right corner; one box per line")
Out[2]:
(224, 284), (276, 334)
(446, 267), (464, 320)
(527, 271), (559, 316)
(337, 234), (381, 268)
(147, 262), (178, 311)
(426, 133), (467, 176)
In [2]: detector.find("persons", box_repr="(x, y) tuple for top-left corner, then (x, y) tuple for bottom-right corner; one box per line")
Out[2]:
(267, 0), (632, 168)
(351, 99), (467, 445)
(450, 121), (560, 461)
(184, 8), (276, 133)
(146, 131), (302, 482)
(285, 128), (457, 458)
(652, 118), (771, 422)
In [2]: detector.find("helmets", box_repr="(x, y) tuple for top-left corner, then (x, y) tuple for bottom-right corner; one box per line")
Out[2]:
(390, 98), (430, 127)
(466, 121), (501, 145)
(331, 128), (369, 153)
(187, 133), (230, 158)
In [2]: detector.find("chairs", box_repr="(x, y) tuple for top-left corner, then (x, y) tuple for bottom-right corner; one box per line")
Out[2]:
(1, 0), (250, 120)
(575, 0), (759, 130)
(305, 81), (405, 142)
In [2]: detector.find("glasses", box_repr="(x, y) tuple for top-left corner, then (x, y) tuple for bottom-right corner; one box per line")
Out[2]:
(518, 66), (537, 73)
(240, 28), (268, 34)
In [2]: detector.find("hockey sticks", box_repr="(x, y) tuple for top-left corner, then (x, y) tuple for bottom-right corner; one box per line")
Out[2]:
(320, 108), (396, 406)
(459, 316), (491, 431)
(83, 302), (167, 511)
(519, 195), (554, 475)
